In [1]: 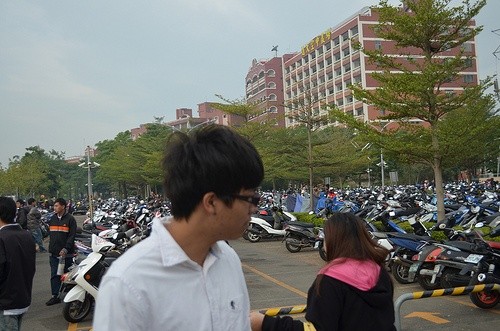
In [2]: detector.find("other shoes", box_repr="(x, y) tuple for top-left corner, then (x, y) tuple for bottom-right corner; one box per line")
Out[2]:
(46, 295), (61, 306)
(40, 249), (47, 253)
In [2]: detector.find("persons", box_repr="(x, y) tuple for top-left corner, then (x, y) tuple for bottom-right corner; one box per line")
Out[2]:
(0, 196), (37, 331)
(269, 182), (336, 213)
(46, 197), (77, 306)
(148, 191), (162, 216)
(249, 212), (397, 331)
(16, 194), (54, 253)
(93, 123), (265, 331)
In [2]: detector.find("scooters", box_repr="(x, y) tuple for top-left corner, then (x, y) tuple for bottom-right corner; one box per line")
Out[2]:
(34, 190), (174, 324)
(240, 177), (500, 309)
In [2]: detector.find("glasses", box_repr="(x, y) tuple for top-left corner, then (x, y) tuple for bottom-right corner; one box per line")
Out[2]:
(232, 192), (261, 205)
